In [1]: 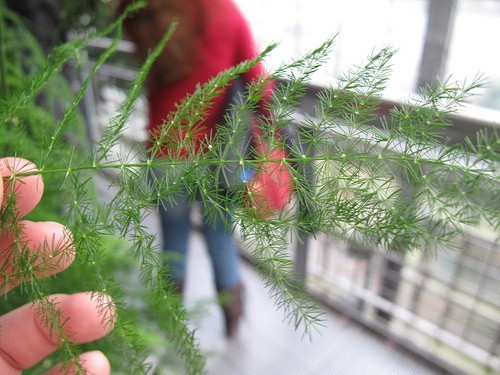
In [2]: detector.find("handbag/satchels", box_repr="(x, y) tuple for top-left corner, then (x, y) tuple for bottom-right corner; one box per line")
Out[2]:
(246, 126), (293, 218)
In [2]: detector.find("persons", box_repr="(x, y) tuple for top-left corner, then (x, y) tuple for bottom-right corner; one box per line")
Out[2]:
(0, 155), (116, 375)
(116, 0), (279, 342)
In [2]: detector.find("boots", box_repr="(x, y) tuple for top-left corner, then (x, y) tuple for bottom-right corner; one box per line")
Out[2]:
(167, 279), (187, 307)
(215, 277), (247, 336)
